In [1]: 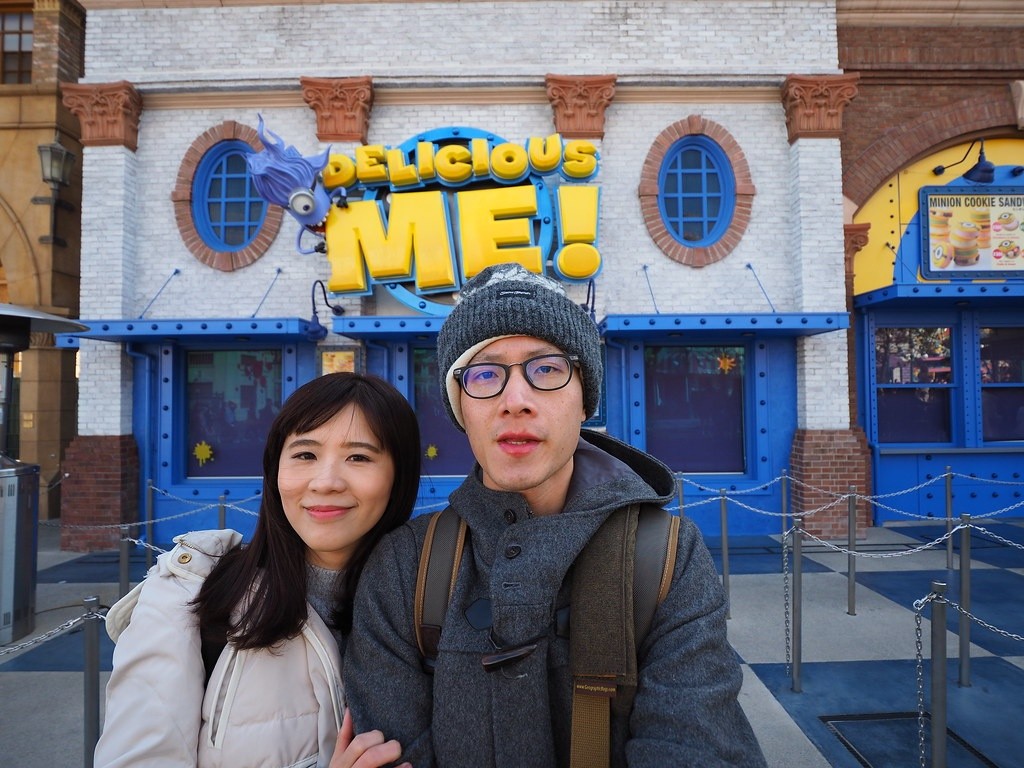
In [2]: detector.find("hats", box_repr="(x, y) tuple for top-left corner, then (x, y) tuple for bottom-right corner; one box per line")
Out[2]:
(436, 263), (605, 435)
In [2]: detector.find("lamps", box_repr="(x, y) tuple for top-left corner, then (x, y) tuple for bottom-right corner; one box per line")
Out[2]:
(932, 137), (995, 184)
(38, 131), (75, 191)
(305, 279), (345, 342)
(580, 279), (604, 337)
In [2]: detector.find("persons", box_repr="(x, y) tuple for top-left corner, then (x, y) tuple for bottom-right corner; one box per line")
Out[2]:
(93, 371), (423, 768)
(342, 263), (768, 768)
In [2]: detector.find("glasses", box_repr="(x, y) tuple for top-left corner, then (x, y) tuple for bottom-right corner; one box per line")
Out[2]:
(452, 353), (580, 399)
